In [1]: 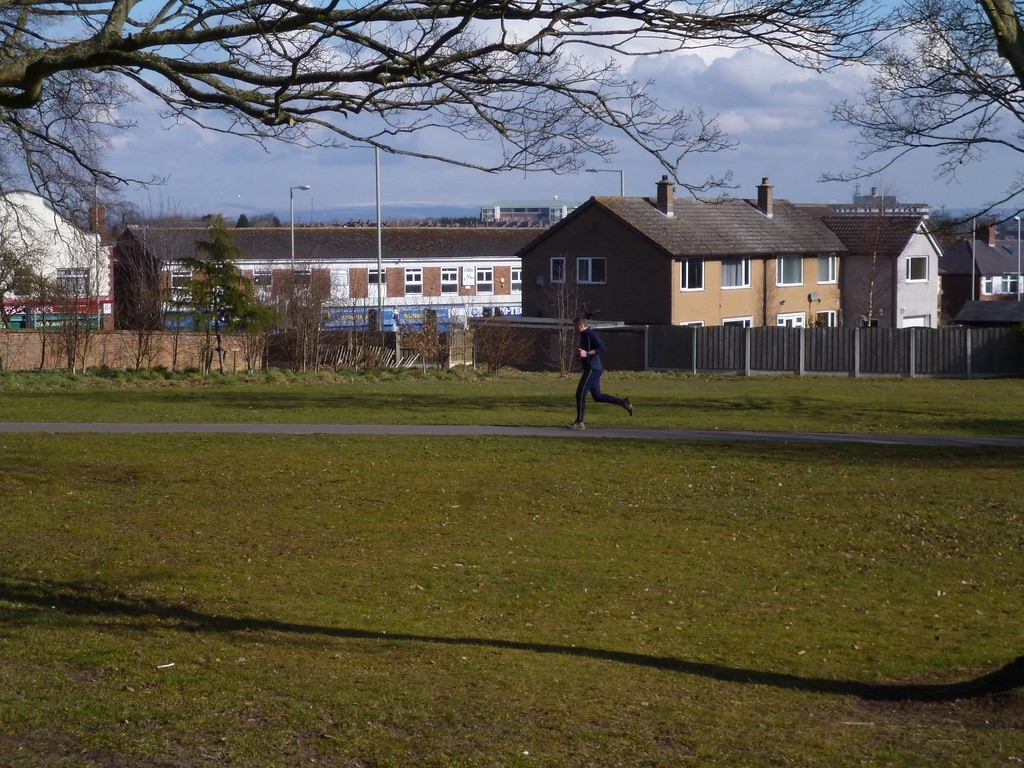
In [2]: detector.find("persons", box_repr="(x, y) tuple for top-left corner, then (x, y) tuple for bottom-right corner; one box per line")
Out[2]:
(567, 318), (633, 430)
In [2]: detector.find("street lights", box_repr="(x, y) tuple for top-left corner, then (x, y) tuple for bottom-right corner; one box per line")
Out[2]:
(586, 169), (624, 196)
(971, 214), (1003, 301)
(290, 184), (310, 269)
(1014, 216), (1020, 302)
(339, 143), (383, 348)
(94, 171), (116, 329)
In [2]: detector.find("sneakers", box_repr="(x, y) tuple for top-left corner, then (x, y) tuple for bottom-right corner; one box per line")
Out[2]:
(622, 396), (633, 416)
(566, 423), (585, 431)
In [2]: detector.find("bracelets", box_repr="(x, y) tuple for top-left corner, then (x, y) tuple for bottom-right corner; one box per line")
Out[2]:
(588, 351), (590, 356)
